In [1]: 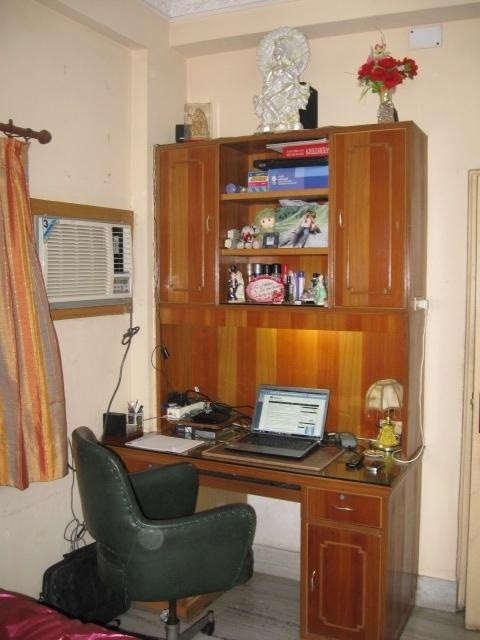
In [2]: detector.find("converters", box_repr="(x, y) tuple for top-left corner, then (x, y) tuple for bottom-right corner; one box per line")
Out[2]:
(161, 347), (170, 359)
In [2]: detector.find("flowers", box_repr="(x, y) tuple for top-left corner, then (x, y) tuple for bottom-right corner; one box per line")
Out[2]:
(349, 30), (418, 101)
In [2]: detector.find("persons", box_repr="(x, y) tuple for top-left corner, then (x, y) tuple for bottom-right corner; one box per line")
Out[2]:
(227, 265), (246, 301)
(257, 42), (297, 124)
(302, 272), (328, 308)
(279, 210), (321, 248)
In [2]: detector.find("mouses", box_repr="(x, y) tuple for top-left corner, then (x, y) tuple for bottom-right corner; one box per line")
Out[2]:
(335, 432), (357, 448)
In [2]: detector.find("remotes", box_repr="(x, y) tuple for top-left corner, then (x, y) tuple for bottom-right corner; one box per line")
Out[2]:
(345, 454), (365, 468)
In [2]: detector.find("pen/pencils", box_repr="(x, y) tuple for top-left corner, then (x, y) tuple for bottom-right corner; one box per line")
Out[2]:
(128, 400), (143, 413)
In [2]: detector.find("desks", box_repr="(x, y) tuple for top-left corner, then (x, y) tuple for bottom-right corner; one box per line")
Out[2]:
(106, 433), (426, 640)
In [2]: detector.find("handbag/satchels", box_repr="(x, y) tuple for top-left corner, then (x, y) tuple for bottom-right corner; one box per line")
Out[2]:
(39, 540), (130, 625)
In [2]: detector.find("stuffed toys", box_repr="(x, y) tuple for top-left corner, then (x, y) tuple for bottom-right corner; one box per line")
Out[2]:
(237, 209), (278, 254)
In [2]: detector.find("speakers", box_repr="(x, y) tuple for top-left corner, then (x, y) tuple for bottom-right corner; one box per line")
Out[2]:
(102, 412), (126, 434)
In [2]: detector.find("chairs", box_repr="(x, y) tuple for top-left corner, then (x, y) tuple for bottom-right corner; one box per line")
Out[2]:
(70, 427), (257, 640)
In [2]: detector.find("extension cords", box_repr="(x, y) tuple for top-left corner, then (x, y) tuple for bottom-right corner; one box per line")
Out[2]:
(167, 402), (205, 421)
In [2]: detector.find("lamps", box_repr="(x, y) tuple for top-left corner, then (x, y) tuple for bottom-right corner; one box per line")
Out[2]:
(363, 379), (407, 458)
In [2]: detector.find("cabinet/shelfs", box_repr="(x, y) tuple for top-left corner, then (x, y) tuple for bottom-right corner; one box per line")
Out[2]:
(156, 123), (424, 462)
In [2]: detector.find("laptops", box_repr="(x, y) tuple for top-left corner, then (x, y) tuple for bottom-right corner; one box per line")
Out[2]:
(224, 383), (331, 459)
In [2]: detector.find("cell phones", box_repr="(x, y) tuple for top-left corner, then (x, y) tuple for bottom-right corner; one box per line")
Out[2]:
(366, 460), (384, 471)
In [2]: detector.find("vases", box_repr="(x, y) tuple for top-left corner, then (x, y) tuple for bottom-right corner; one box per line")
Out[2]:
(377, 93), (395, 121)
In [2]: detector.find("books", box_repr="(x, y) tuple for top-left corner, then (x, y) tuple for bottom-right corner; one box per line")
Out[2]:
(252, 138), (330, 170)
(123, 432), (206, 456)
